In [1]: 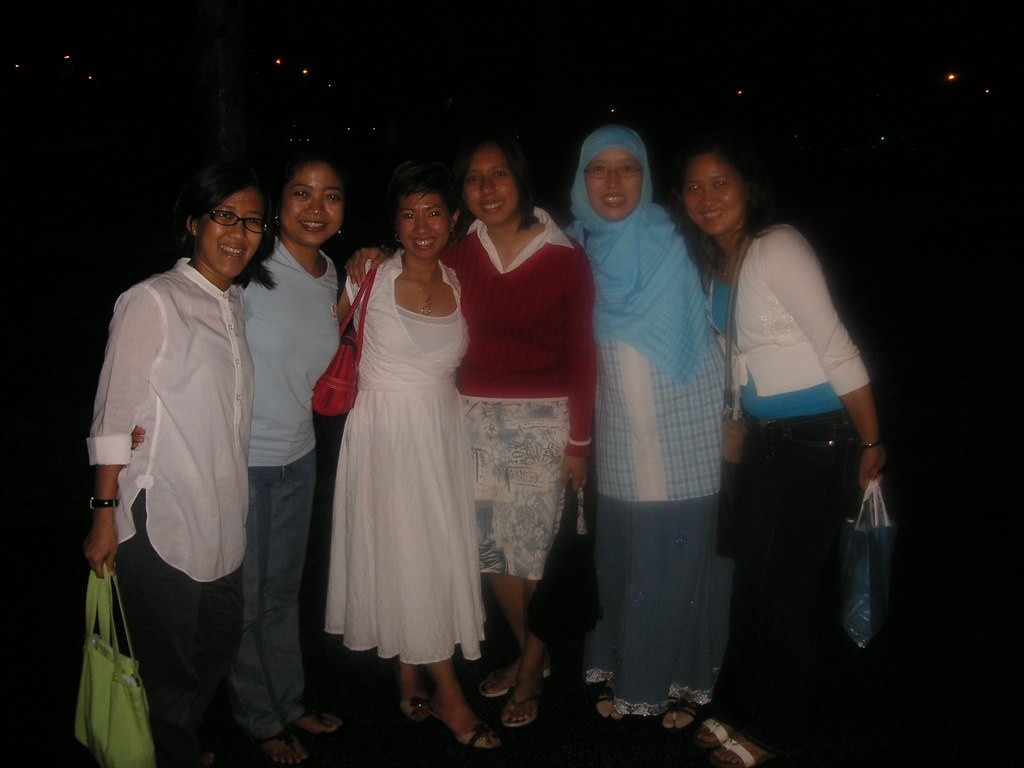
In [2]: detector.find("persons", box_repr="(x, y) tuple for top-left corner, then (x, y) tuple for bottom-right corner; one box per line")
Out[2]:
(85, 162), (274, 768)
(346, 138), (596, 729)
(132, 152), (344, 768)
(326, 159), (504, 749)
(678, 142), (885, 768)
(561, 126), (731, 729)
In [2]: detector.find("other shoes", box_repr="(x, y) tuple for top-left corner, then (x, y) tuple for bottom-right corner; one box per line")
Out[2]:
(199, 752), (214, 768)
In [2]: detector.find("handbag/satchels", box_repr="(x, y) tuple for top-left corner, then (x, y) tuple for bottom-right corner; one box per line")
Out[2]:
(312, 258), (376, 416)
(837, 481), (898, 647)
(528, 479), (600, 639)
(75, 560), (156, 768)
(716, 414), (747, 558)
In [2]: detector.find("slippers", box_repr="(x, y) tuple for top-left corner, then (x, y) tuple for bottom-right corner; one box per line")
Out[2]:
(502, 688), (540, 727)
(479, 661), (552, 698)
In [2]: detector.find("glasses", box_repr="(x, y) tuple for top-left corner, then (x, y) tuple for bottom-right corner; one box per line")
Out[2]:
(584, 164), (643, 179)
(202, 209), (269, 233)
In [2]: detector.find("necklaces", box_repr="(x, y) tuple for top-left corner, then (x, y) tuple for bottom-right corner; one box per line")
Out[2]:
(724, 272), (727, 276)
(418, 288), (432, 315)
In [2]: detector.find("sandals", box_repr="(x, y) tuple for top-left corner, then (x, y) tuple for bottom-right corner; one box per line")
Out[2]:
(709, 730), (776, 768)
(288, 707), (343, 736)
(693, 717), (739, 749)
(393, 664), (431, 722)
(659, 702), (698, 733)
(249, 728), (308, 767)
(595, 682), (627, 726)
(428, 697), (501, 749)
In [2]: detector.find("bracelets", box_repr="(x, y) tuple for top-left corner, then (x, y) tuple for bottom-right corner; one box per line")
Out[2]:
(863, 437), (883, 448)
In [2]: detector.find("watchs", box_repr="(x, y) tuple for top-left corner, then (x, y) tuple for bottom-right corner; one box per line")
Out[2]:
(89, 497), (118, 510)
